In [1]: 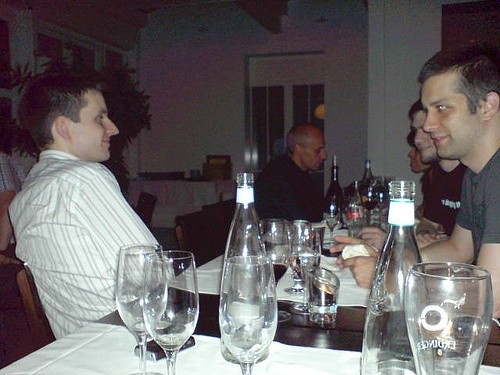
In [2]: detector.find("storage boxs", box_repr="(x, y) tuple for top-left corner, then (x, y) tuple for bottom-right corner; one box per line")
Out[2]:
(202, 155), (232, 181)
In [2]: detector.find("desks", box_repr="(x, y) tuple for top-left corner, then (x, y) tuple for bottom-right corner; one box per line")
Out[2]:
(128, 180), (235, 229)
(0, 231), (500, 375)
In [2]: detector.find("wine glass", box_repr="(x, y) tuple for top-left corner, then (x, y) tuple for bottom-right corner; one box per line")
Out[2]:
(223, 256), (278, 375)
(143, 251), (200, 375)
(260, 217), (322, 323)
(115, 244), (168, 375)
(323, 199), (340, 243)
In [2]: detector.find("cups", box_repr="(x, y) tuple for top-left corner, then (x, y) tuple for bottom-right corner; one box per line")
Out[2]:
(404, 261), (494, 375)
(189, 169), (200, 178)
(304, 266), (340, 324)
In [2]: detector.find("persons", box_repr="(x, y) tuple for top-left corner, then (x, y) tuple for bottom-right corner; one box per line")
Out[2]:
(254, 123), (328, 222)
(329, 40), (500, 318)
(0, 151), (28, 254)
(8, 72), (176, 341)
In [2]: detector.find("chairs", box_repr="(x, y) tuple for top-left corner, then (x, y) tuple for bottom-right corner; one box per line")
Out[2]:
(175, 208), (228, 268)
(201, 198), (235, 231)
(138, 192), (157, 226)
(17, 270), (56, 349)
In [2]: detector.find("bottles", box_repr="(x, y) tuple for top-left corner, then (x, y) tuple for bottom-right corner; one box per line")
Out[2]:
(349, 180), (361, 206)
(327, 166), (341, 218)
(360, 180), (435, 375)
(218, 172), (270, 364)
(360, 159), (374, 187)
